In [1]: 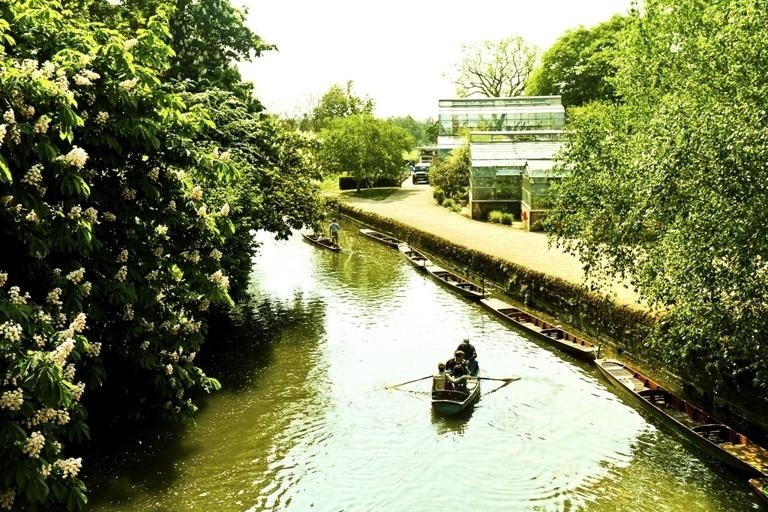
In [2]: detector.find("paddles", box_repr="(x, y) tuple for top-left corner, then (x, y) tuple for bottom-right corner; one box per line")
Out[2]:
(468, 376), (521, 382)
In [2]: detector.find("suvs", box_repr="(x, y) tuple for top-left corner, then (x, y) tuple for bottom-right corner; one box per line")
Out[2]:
(412, 162), (431, 185)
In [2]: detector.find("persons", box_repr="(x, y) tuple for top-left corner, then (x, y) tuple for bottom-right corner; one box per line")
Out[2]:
(328, 217), (340, 250)
(453, 339), (479, 376)
(450, 364), (470, 397)
(445, 350), (472, 382)
(432, 363), (470, 392)
(313, 219), (325, 237)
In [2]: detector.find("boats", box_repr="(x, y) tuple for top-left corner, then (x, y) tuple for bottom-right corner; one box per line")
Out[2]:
(424, 258), (493, 299)
(477, 294), (604, 359)
(300, 228), (342, 253)
(397, 241), (434, 268)
(593, 359), (768, 481)
(430, 366), (481, 417)
(358, 226), (408, 247)
